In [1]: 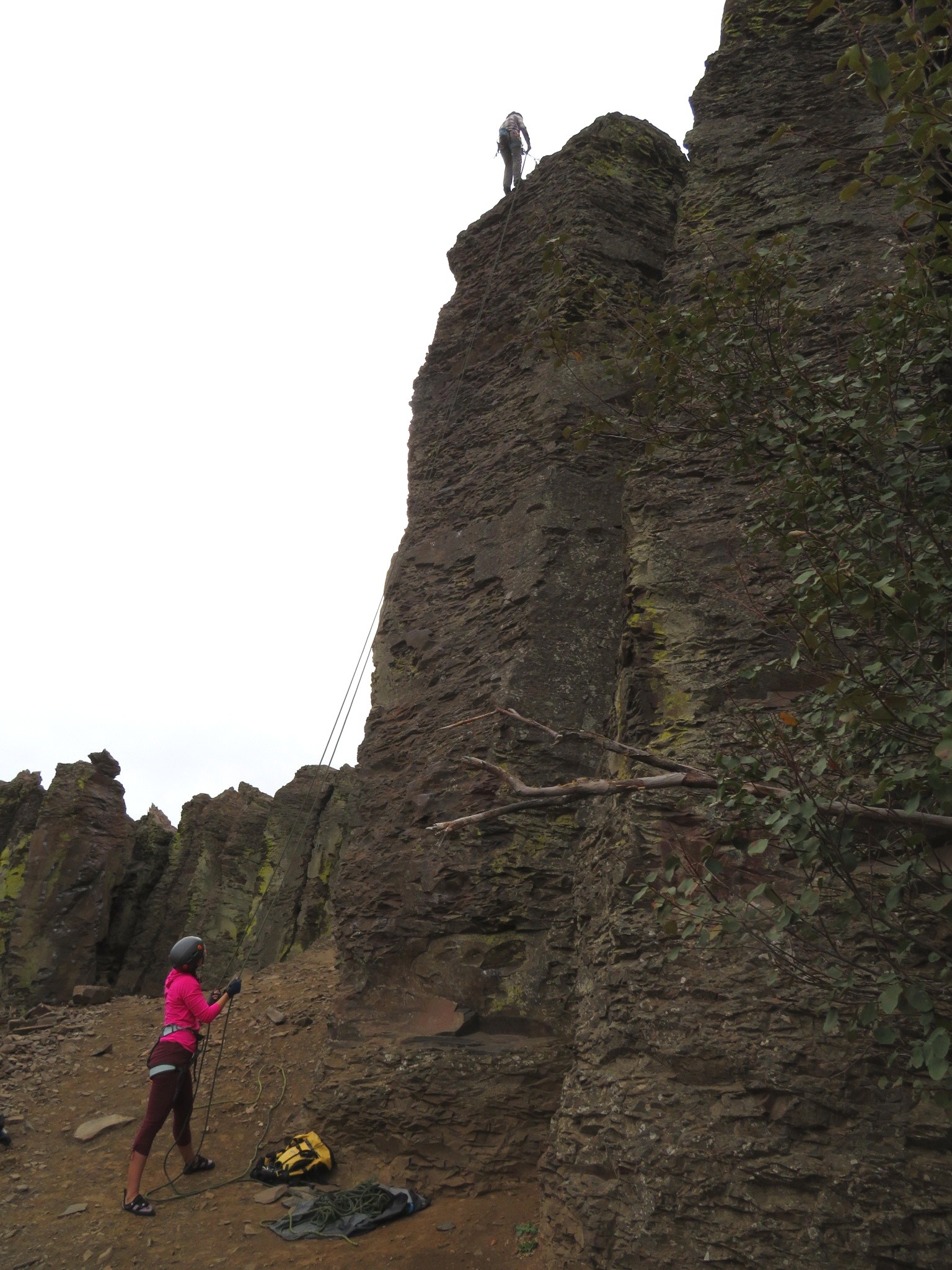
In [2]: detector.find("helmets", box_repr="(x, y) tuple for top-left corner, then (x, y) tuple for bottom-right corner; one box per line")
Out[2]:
(169, 936), (205, 970)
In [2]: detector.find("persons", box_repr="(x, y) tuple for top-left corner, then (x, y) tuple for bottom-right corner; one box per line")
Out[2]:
(499, 112), (531, 195)
(121, 935), (241, 1216)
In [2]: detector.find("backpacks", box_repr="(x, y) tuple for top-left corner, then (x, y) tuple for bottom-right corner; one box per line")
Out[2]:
(250, 1131), (337, 1186)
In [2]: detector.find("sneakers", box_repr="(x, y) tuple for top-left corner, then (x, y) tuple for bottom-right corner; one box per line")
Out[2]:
(122, 1188), (156, 1217)
(183, 1155), (216, 1176)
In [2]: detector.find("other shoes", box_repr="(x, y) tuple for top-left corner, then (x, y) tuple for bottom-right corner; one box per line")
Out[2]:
(514, 178), (523, 187)
(504, 187), (511, 196)
